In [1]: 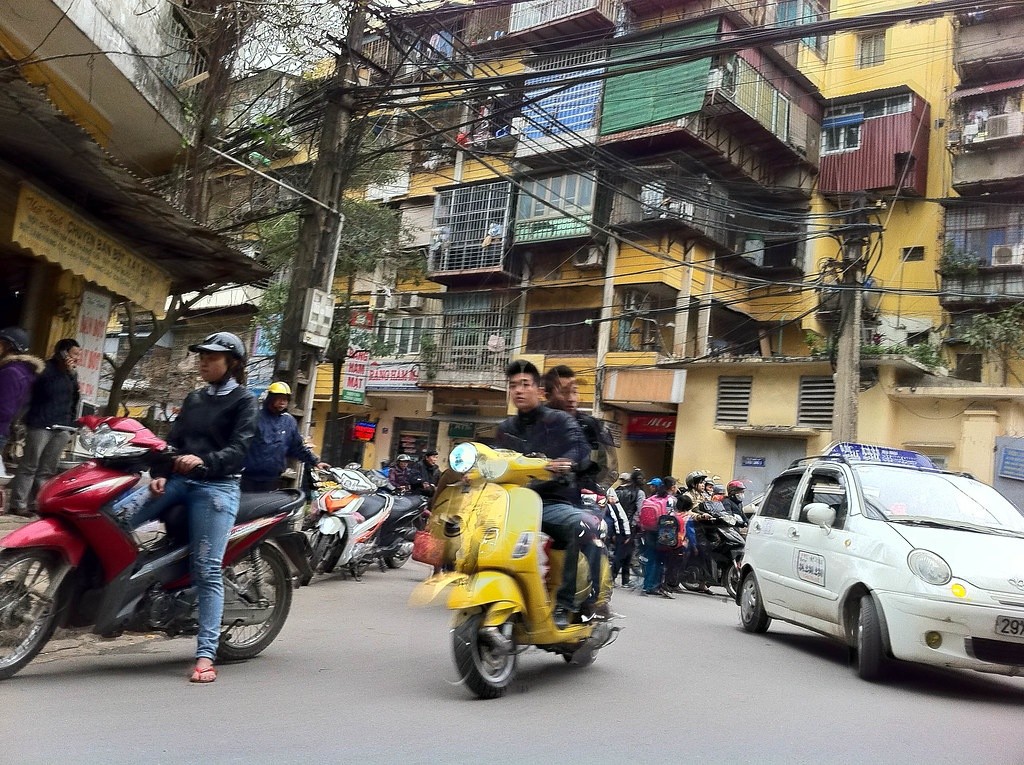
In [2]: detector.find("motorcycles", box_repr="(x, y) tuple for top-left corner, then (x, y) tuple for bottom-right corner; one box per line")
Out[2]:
(311, 462), (441, 584)
(0, 409), (307, 680)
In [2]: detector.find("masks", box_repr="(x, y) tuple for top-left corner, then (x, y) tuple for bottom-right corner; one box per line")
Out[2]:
(735, 492), (745, 502)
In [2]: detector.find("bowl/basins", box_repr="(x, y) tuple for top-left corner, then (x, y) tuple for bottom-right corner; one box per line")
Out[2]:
(0, 473), (15, 489)
(58, 461), (85, 470)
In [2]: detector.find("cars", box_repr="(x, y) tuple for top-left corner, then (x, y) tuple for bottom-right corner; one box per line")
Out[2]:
(735, 442), (1023, 681)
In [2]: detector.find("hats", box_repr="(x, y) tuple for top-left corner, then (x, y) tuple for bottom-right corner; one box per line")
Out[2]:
(646, 477), (662, 486)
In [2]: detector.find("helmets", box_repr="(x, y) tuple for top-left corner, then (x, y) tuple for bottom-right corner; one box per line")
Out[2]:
(686, 471), (708, 487)
(397, 454), (410, 462)
(714, 485), (726, 493)
(0, 327), (31, 354)
(187, 330), (248, 366)
(269, 382), (291, 396)
(706, 478), (715, 485)
(727, 481), (746, 497)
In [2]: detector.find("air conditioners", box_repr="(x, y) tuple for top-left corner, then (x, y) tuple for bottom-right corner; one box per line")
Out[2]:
(992, 243), (1024, 266)
(624, 289), (650, 314)
(374, 289), (398, 310)
(572, 245), (602, 270)
(986, 111), (1023, 142)
(396, 290), (423, 312)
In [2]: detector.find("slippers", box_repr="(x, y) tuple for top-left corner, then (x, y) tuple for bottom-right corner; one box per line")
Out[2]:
(190, 664), (215, 683)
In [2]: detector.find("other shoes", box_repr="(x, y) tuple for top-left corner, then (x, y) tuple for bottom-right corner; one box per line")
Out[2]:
(552, 606), (569, 629)
(661, 585), (675, 598)
(579, 606), (593, 624)
(663, 582), (683, 593)
(10, 505), (36, 517)
(623, 579), (639, 588)
(700, 587), (716, 594)
(643, 588), (663, 597)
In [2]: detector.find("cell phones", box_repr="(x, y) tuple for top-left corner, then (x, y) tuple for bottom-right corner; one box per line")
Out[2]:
(60, 350), (69, 361)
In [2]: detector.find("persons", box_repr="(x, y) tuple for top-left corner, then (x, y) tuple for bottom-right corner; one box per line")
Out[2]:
(0, 328), (45, 454)
(495, 359), (619, 631)
(607, 468), (749, 599)
(379, 450), (443, 490)
(115, 331), (256, 683)
(241, 382), (330, 493)
(8, 339), (81, 517)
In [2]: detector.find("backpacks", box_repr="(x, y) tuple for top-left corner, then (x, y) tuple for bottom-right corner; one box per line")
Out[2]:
(614, 485), (642, 522)
(657, 514), (680, 546)
(640, 493), (667, 531)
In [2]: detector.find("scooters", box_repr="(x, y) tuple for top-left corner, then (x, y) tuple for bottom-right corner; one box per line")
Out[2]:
(448, 439), (624, 701)
(609, 507), (751, 603)
